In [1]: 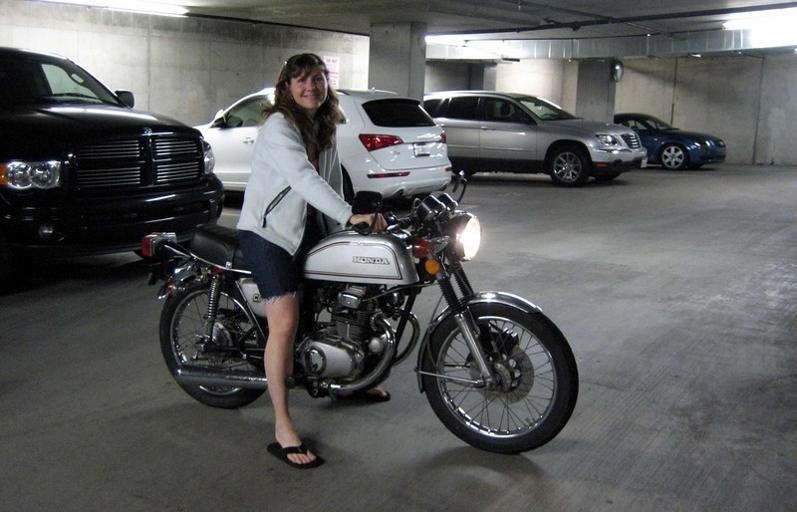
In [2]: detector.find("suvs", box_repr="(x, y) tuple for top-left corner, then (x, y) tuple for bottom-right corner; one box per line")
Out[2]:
(191, 87), (453, 204)
(0, 45), (224, 269)
(423, 89), (648, 186)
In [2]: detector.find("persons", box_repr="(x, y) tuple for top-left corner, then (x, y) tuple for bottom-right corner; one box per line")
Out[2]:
(235, 51), (393, 469)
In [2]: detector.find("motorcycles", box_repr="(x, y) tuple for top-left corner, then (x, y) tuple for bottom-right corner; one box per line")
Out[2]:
(141, 164), (579, 454)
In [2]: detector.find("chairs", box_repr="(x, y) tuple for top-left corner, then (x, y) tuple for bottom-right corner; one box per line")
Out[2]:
(500, 102), (511, 118)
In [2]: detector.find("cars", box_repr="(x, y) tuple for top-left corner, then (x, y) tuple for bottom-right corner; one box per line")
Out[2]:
(614, 113), (726, 170)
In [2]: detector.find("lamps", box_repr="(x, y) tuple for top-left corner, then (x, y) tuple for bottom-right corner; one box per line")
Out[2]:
(612, 59), (625, 81)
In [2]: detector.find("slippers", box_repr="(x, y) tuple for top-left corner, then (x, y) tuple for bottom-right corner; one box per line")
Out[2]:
(267, 440), (324, 469)
(337, 386), (391, 402)
